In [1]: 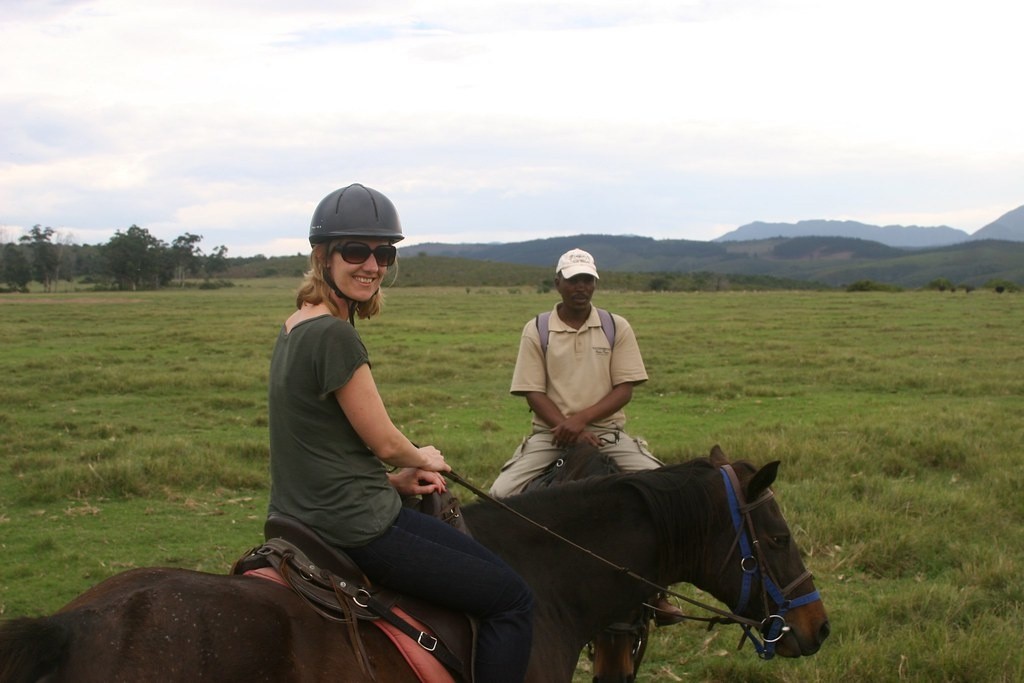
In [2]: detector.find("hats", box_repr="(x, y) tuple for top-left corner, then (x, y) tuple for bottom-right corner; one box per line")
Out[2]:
(556, 248), (600, 280)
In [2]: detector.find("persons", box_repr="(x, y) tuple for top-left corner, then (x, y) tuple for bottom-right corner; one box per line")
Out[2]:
(268, 184), (531, 683)
(490, 248), (667, 499)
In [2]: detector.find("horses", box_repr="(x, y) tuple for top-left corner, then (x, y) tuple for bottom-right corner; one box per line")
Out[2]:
(0, 443), (832, 683)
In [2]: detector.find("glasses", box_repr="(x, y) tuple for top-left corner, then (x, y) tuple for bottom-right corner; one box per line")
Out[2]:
(334, 241), (396, 266)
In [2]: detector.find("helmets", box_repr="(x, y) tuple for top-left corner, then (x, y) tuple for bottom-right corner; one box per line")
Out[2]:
(309, 183), (405, 241)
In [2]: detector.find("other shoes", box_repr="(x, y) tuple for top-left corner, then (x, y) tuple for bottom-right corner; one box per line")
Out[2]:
(652, 595), (685, 626)
